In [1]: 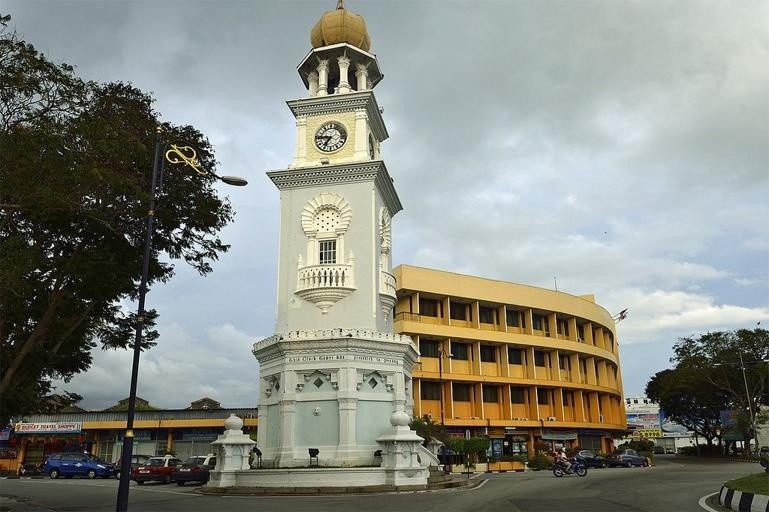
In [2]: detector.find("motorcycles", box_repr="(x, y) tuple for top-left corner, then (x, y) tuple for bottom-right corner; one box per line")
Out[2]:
(552, 455), (587, 477)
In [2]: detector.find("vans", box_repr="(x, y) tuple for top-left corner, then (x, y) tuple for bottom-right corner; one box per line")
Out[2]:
(115, 454), (155, 480)
(43, 450), (115, 480)
(653, 446), (664, 454)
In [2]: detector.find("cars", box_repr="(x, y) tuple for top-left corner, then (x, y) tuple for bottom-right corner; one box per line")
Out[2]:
(130, 454), (183, 485)
(606, 448), (651, 468)
(567, 448), (608, 469)
(170, 453), (218, 486)
(20, 464), (41, 475)
(735, 443), (768, 456)
(665, 447), (675, 454)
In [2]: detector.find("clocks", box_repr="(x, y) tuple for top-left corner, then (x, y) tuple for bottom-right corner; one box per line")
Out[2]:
(315, 124), (347, 152)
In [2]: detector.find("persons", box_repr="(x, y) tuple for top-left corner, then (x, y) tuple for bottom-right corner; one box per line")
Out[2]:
(559, 446), (575, 477)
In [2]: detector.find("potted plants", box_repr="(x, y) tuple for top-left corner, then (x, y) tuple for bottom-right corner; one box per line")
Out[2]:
(475, 455), (525, 472)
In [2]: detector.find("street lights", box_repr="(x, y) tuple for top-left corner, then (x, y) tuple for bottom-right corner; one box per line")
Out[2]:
(438, 347), (454, 456)
(114, 123), (250, 511)
